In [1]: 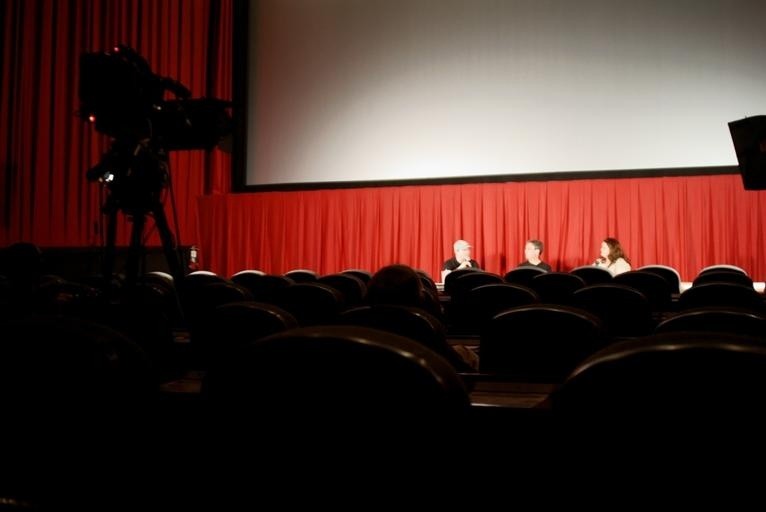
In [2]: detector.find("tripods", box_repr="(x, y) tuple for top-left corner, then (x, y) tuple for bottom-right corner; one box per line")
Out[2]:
(101, 192), (185, 280)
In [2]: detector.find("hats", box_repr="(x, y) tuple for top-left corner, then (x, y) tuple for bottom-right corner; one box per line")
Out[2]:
(453, 239), (472, 252)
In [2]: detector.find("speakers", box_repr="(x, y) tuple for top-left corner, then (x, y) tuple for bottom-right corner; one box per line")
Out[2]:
(728, 115), (766, 190)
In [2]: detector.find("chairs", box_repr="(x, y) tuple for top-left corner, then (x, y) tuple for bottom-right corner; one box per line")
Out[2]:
(0, 258), (766, 512)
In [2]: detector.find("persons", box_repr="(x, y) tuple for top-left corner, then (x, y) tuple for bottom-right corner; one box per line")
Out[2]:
(517, 240), (551, 273)
(440, 239), (479, 284)
(592, 237), (632, 278)
(364, 264), (482, 395)
